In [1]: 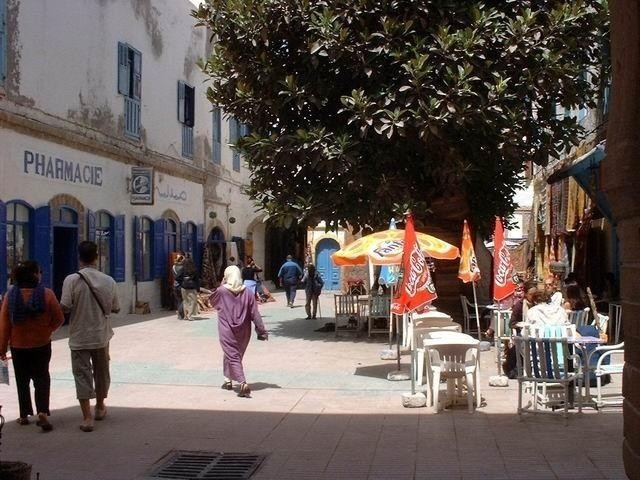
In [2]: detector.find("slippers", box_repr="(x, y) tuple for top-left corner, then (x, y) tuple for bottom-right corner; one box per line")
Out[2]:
(16, 418), (30, 425)
(36, 419), (53, 432)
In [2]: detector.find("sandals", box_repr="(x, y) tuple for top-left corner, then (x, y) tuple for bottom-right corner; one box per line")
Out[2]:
(238, 381), (250, 398)
(222, 381), (232, 390)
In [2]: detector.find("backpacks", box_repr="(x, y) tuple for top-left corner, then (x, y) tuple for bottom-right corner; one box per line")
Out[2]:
(502, 344), (524, 379)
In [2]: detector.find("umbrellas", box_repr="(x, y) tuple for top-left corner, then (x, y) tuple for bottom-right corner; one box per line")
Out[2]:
(492, 215), (519, 376)
(331, 229), (462, 370)
(458, 218), (483, 342)
(378, 217), (401, 350)
(390, 209), (438, 395)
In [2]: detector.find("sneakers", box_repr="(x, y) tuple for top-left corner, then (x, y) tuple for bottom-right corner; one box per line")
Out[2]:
(94, 404), (107, 420)
(80, 418), (93, 431)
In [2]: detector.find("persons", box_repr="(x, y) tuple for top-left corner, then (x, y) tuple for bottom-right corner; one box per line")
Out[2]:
(278, 255), (303, 308)
(169, 252), (267, 321)
(484, 272), (620, 381)
(0, 260), (65, 432)
(301, 264), (323, 319)
(58, 241), (120, 432)
(209, 265), (268, 398)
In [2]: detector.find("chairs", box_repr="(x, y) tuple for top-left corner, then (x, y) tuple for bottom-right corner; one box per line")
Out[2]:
(403, 292), (625, 427)
(333, 294), (364, 336)
(367, 293), (399, 337)
